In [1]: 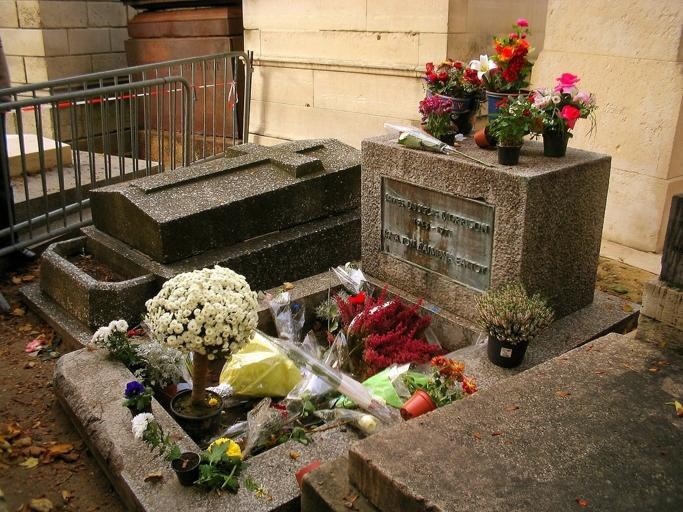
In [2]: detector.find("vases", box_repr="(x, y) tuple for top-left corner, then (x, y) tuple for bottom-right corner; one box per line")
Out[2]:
(126, 404), (152, 416)
(171, 451), (202, 487)
(488, 331), (530, 369)
(400, 388), (437, 420)
(470, 126), (573, 166)
(170, 389), (224, 441)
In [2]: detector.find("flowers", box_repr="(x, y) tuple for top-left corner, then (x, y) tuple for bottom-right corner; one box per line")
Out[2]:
(120, 381), (154, 410)
(279, 302), (304, 320)
(421, 19), (597, 142)
(355, 414), (382, 435)
(91, 319), (129, 350)
(420, 355), (479, 409)
(472, 281), (555, 347)
(192, 438), (243, 495)
(221, 403), (289, 489)
(145, 265), (260, 406)
(131, 412), (189, 468)
(333, 282), (443, 378)
